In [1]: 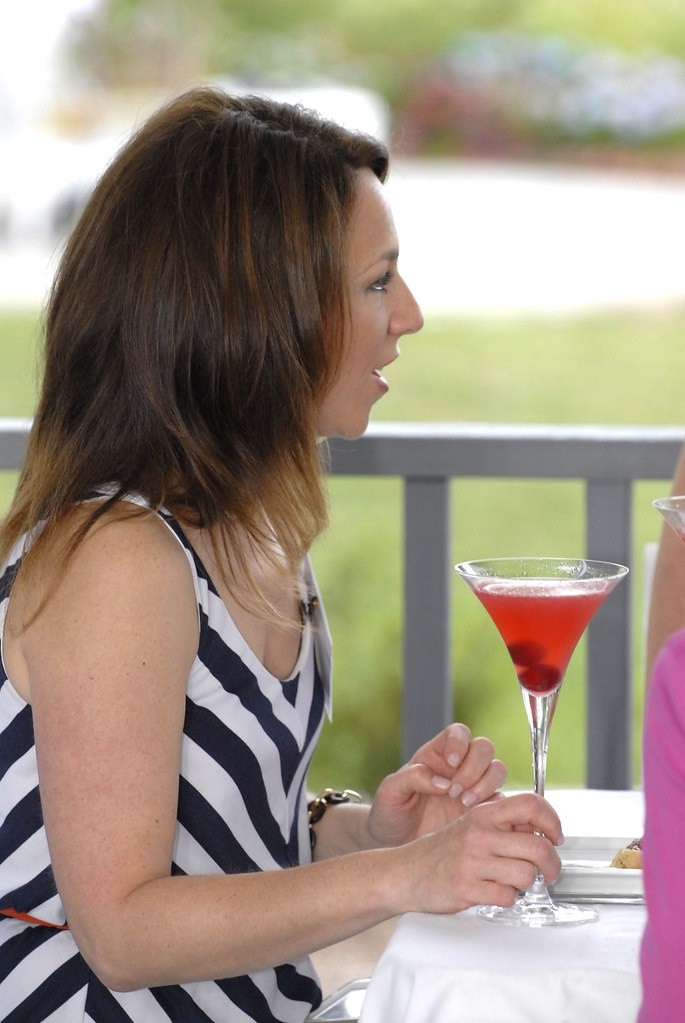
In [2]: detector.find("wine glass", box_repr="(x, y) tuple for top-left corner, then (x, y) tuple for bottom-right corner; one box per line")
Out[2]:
(454, 557), (628, 927)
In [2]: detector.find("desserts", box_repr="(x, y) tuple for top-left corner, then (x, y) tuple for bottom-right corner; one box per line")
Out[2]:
(611, 836), (643, 868)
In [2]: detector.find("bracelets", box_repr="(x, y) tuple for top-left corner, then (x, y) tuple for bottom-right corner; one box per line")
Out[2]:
(308, 788), (363, 848)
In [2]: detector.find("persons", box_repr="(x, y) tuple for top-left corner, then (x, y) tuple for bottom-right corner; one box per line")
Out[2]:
(0, 89), (564, 1022)
(633, 450), (685, 1023)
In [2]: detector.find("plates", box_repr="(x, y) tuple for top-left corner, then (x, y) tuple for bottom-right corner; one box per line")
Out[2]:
(550, 837), (645, 896)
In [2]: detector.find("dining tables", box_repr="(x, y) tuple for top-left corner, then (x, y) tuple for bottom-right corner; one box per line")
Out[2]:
(354, 787), (650, 1021)
